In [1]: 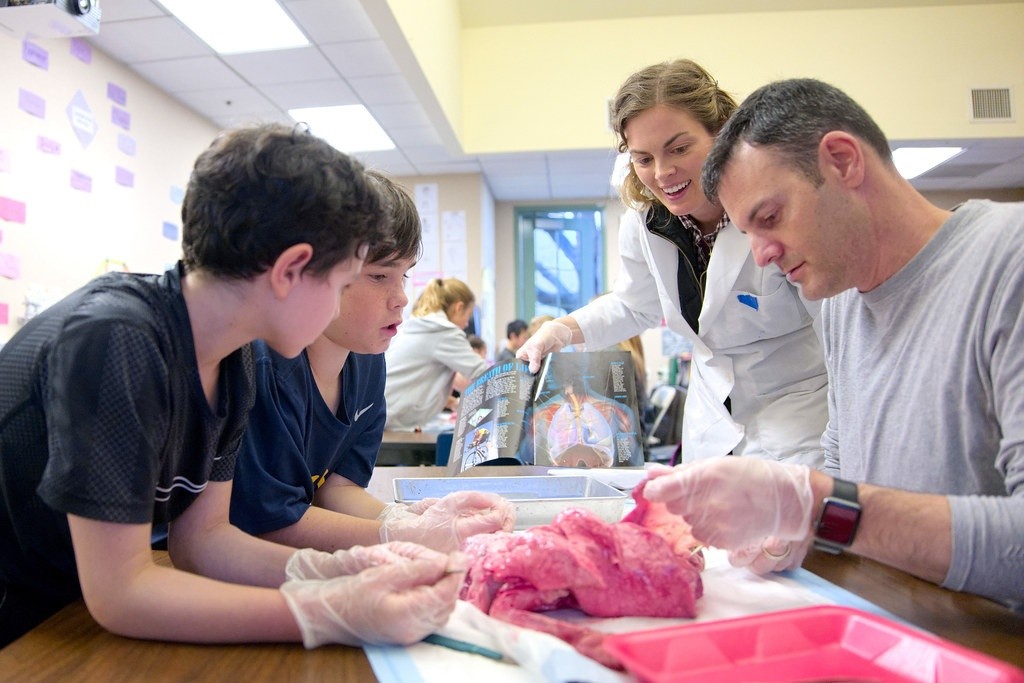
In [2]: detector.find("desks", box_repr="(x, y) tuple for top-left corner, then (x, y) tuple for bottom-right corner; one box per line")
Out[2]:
(0, 464), (1024, 683)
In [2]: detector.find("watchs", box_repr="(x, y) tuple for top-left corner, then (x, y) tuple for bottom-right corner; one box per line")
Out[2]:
(814, 478), (863, 556)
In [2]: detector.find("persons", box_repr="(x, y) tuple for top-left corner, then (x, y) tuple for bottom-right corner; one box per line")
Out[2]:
(383, 277), (490, 431)
(633, 79), (1024, 611)
(517, 57), (828, 469)
(442, 314), (647, 444)
(145, 163), (520, 558)
(0, 122), (465, 656)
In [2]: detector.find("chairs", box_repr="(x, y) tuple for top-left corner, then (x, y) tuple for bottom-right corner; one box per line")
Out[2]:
(643, 385), (679, 446)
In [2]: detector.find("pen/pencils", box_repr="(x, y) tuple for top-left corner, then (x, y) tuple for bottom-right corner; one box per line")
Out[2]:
(422, 634), (519, 666)
